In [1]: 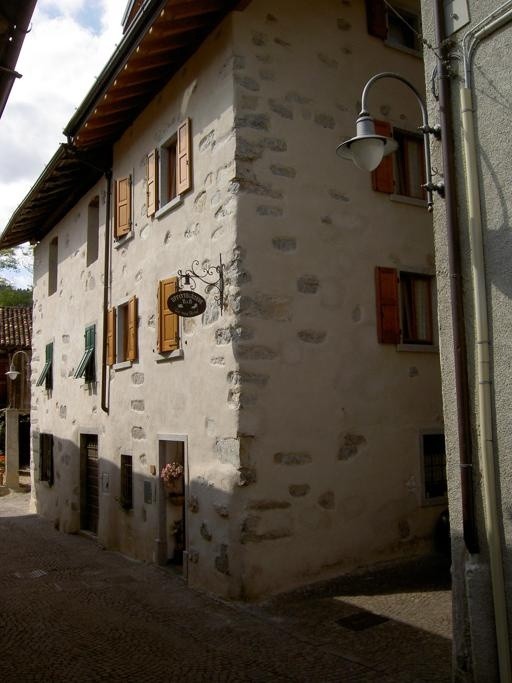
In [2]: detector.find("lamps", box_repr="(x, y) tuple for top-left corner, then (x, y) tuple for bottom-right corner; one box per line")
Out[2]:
(336, 71), (445, 213)
(4, 351), (30, 381)
